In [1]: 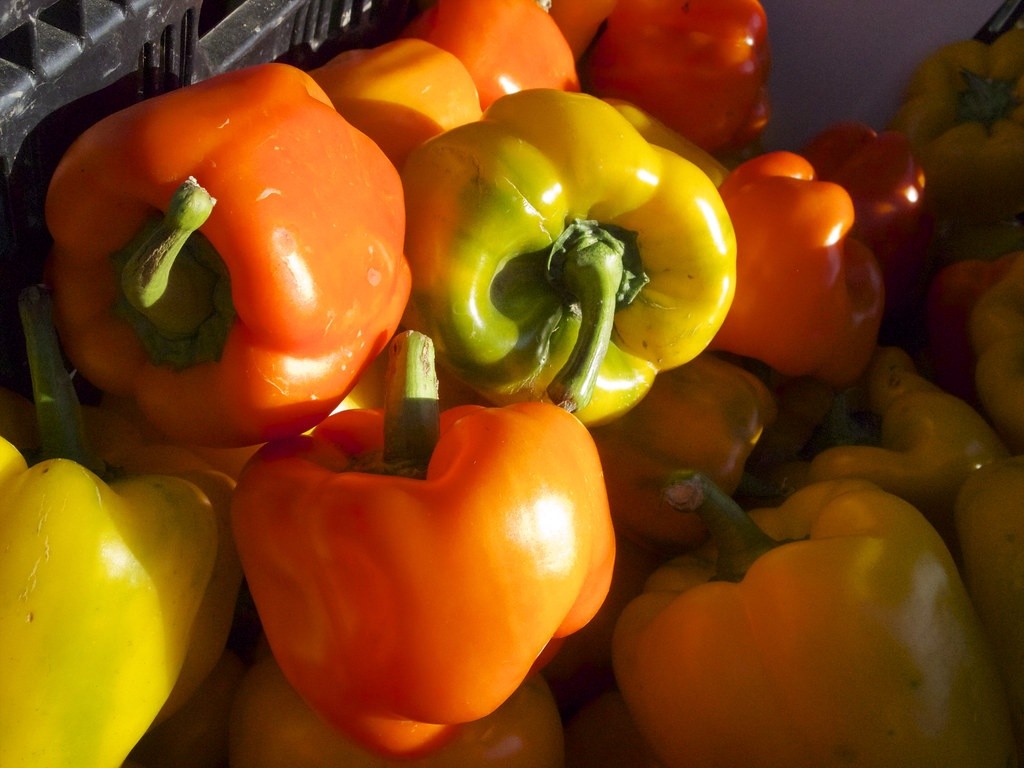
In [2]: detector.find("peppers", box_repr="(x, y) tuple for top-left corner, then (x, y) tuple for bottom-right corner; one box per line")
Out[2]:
(1, 2), (1024, 768)
